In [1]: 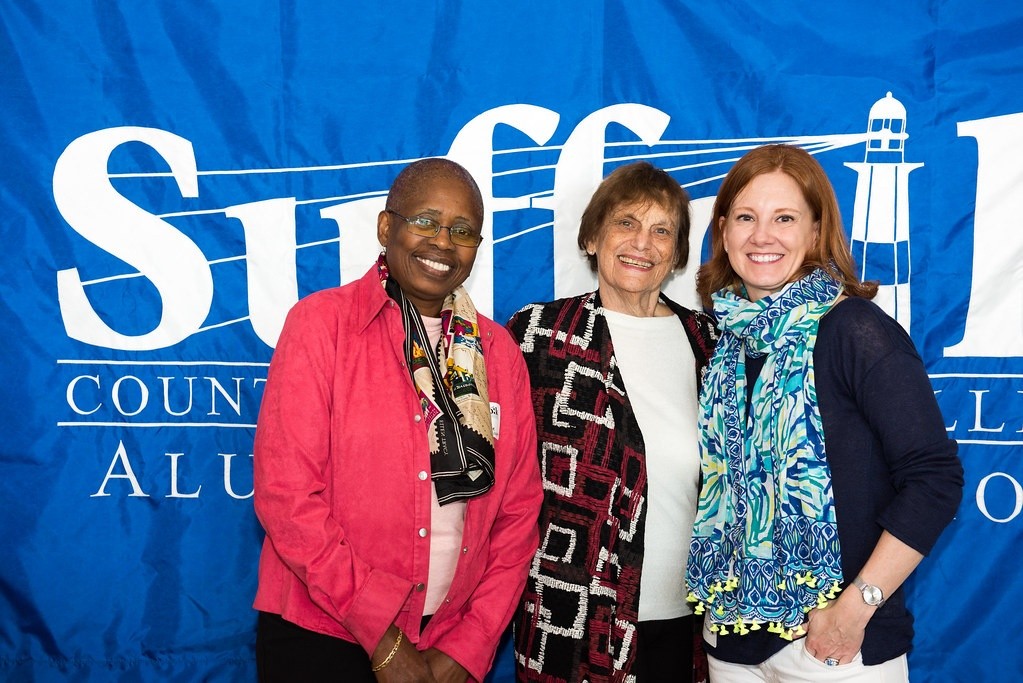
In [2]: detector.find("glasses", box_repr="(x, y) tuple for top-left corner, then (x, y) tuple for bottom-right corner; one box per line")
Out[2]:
(387, 209), (483, 247)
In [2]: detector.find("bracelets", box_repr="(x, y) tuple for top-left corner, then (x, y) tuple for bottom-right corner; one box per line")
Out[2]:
(372, 629), (403, 672)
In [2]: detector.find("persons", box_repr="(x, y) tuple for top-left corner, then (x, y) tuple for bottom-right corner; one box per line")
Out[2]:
(684, 144), (966, 683)
(251, 156), (545, 683)
(503, 160), (719, 682)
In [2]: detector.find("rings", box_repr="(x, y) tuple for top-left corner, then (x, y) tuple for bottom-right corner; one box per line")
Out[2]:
(824, 658), (839, 666)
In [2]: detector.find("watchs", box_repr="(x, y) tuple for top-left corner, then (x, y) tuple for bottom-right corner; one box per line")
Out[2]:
(853, 576), (886, 608)
